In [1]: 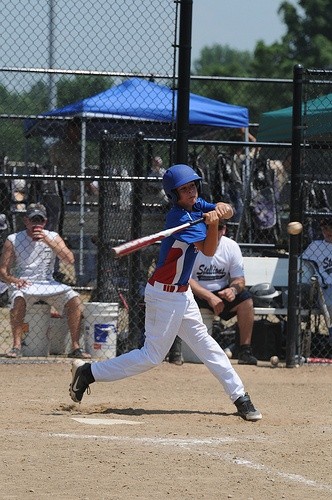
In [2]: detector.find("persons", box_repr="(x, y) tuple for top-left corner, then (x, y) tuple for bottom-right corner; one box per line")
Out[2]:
(169, 219), (258, 365)
(298, 219), (332, 346)
(0, 202), (93, 359)
(68, 164), (263, 420)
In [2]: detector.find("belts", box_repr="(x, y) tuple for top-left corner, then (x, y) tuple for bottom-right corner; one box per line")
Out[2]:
(148, 278), (189, 292)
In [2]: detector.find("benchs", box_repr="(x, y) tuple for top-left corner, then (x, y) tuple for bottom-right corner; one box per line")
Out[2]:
(183, 257), (321, 364)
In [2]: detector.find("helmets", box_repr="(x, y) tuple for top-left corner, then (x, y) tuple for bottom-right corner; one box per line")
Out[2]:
(163, 164), (201, 204)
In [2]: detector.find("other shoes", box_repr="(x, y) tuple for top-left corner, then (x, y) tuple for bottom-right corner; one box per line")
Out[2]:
(68, 347), (92, 359)
(6, 348), (23, 358)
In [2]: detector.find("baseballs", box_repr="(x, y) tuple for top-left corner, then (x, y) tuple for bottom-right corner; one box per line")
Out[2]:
(287, 222), (302, 234)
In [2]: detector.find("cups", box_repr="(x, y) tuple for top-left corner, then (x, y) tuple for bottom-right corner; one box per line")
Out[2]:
(32, 224), (43, 239)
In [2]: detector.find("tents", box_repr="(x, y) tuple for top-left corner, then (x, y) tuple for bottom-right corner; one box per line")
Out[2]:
(249, 94), (332, 147)
(22, 77), (251, 276)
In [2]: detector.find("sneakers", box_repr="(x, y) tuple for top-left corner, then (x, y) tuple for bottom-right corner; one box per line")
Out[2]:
(238, 345), (257, 365)
(169, 337), (182, 365)
(234, 392), (263, 421)
(69, 359), (95, 402)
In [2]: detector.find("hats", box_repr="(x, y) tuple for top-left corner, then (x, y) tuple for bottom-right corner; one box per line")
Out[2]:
(25, 203), (48, 221)
(206, 219), (227, 230)
(319, 216), (332, 225)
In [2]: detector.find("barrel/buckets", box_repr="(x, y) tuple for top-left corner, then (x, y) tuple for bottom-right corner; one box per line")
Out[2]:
(83, 303), (118, 358)
(22, 303), (50, 356)
(181, 309), (213, 365)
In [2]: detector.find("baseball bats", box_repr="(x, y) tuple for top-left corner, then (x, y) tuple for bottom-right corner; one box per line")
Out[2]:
(111, 216), (207, 259)
(301, 275), (332, 363)
